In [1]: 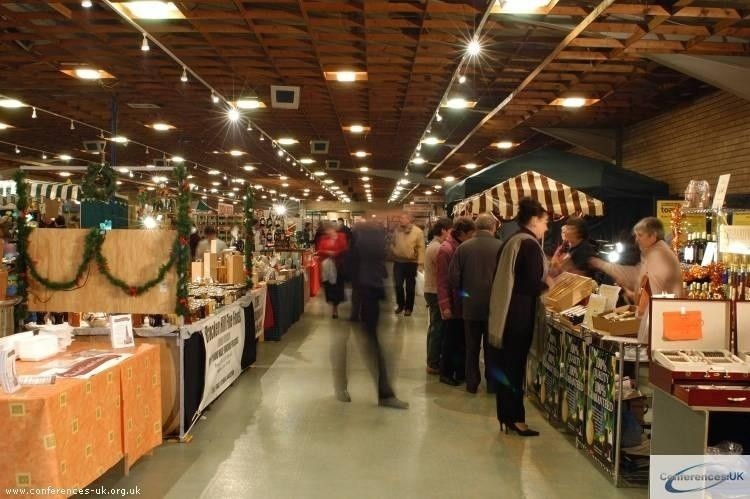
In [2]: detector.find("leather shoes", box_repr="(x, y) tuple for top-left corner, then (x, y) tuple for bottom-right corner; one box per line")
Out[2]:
(440, 376), (458, 385)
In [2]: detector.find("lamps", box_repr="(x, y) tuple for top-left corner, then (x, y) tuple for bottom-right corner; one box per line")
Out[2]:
(141, 34), (149, 51)
(181, 66), (187, 82)
(210, 89), (219, 103)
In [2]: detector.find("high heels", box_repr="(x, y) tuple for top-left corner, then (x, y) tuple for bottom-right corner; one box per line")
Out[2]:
(497, 415), (528, 432)
(504, 422), (539, 436)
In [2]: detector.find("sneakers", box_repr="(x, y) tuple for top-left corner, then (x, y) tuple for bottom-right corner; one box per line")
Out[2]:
(378, 395), (409, 408)
(404, 310), (411, 316)
(427, 365), (441, 375)
(335, 390), (351, 403)
(394, 306), (403, 313)
(466, 382), (478, 394)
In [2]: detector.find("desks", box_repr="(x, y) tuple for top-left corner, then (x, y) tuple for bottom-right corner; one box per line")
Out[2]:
(541, 315), (650, 490)
(54, 289), (253, 444)
(645, 383), (750, 457)
(0, 343), (158, 495)
(262, 270), (306, 344)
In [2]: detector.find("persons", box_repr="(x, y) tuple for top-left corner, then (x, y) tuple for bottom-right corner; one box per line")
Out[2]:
(338, 217), (350, 231)
(553, 225), (566, 261)
(329, 220), (409, 411)
(388, 211), (425, 317)
(435, 216), (475, 383)
(445, 213), (505, 396)
(54, 215), (67, 229)
(564, 216), (599, 283)
(317, 222), (348, 319)
(587, 217), (683, 298)
(195, 225), (228, 259)
(425, 217), (452, 374)
(304, 222), (312, 231)
(485, 197), (552, 437)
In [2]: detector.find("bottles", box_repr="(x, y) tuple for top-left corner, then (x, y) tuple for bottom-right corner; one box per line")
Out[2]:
(704, 282), (708, 294)
(694, 283), (701, 299)
(681, 282), (688, 298)
(684, 231), (716, 265)
(693, 282), (697, 293)
(707, 283), (713, 299)
(562, 305), (588, 325)
(713, 288), (724, 299)
(687, 284), (694, 299)
(699, 284), (707, 299)
(729, 263), (750, 300)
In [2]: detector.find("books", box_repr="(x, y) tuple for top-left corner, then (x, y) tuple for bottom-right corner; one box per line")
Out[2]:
(109, 313), (136, 350)
